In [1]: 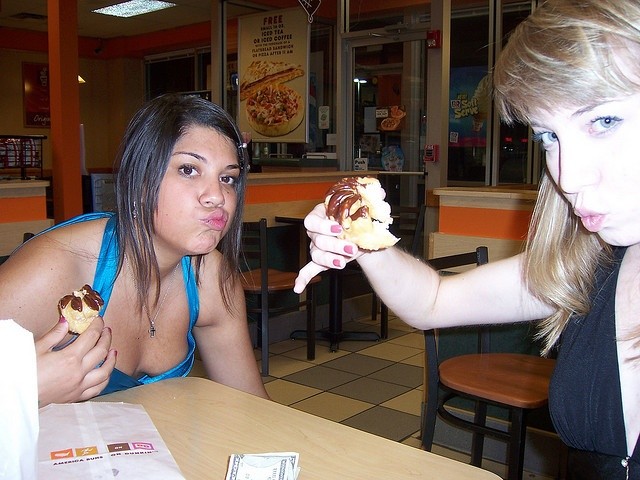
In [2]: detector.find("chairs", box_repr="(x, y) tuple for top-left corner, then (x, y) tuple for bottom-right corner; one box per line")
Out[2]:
(414, 246), (558, 480)
(343, 204), (426, 339)
(239, 218), (323, 377)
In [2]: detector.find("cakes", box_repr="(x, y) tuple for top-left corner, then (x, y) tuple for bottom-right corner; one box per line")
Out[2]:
(56, 283), (105, 336)
(321, 175), (401, 253)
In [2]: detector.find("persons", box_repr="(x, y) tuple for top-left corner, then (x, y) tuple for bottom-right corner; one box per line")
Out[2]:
(293, 0), (640, 480)
(0, 90), (271, 411)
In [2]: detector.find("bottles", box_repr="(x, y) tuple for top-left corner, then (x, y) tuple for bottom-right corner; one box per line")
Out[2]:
(262, 142), (270, 160)
(252, 142), (262, 158)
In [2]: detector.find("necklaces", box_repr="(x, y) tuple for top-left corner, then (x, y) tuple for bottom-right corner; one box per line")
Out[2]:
(125, 252), (181, 336)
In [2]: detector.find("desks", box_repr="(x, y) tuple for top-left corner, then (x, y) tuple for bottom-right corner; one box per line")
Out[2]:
(38, 377), (503, 480)
